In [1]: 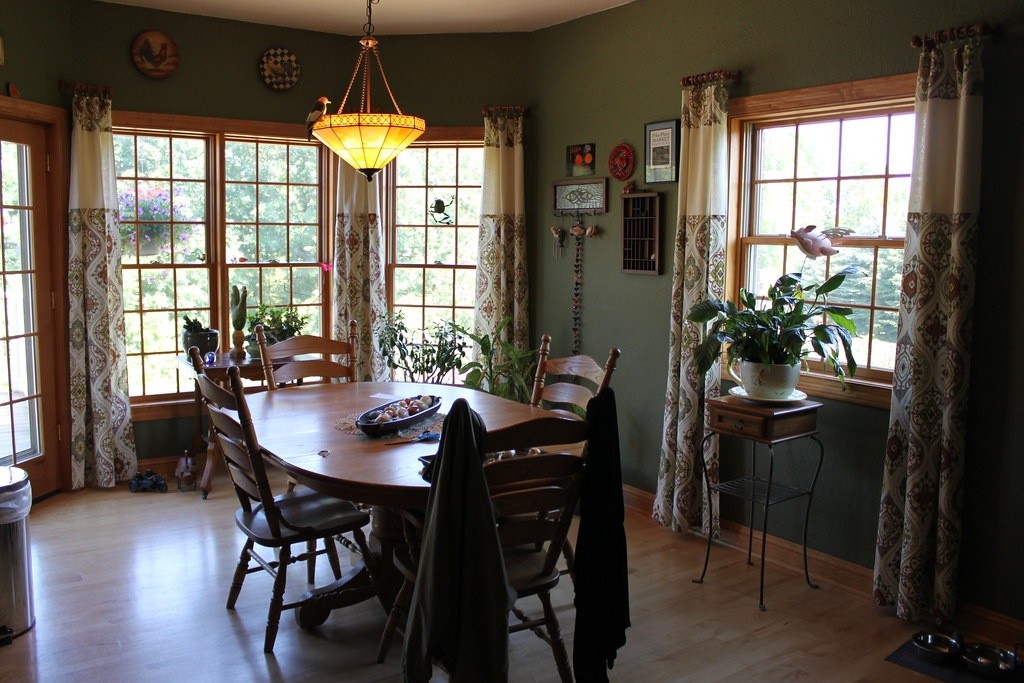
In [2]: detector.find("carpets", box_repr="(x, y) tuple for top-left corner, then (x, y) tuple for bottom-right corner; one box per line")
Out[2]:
(882, 630), (1024, 683)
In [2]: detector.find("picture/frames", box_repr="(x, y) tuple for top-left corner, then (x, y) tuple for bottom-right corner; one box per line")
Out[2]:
(551, 177), (608, 216)
(644, 119), (679, 183)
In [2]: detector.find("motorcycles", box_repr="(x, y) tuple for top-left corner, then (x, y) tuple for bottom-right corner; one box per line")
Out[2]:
(129, 468), (168, 494)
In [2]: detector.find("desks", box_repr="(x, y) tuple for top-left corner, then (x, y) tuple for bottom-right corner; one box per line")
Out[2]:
(177, 352), (320, 500)
(238, 381), (579, 630)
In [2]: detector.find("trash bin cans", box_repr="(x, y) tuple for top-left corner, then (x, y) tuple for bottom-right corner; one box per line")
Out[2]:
(0, 465), (37, 645)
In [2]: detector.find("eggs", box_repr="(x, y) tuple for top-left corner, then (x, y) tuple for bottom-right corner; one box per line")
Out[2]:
(369, 396), (432, 423)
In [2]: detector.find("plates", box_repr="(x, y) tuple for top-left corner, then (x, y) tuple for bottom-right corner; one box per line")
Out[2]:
(358, 396), (442, 435)
(418, 445), (547, 469)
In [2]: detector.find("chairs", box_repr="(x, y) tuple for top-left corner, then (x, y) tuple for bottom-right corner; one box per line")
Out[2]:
(375, 386), (632, 683)
(188, 346), (370, 653)
(529, 335), (621, 609)
(254, 317), (357, 492)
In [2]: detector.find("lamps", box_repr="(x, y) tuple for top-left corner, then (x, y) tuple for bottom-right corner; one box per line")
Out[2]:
(310, 0), (425, 182)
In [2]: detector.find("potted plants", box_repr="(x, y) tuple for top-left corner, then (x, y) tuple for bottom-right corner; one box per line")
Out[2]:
(688, 272), (859, 400)
(245, 304), (308, 360)
(182, 315), (220, 365)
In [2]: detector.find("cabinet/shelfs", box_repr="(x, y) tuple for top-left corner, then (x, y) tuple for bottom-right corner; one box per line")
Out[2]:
(691, 392), (825, 611)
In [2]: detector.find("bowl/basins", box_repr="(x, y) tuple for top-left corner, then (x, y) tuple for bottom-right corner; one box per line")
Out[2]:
(912, 632), (959, 658)
(963, 642), (1020, 677)
